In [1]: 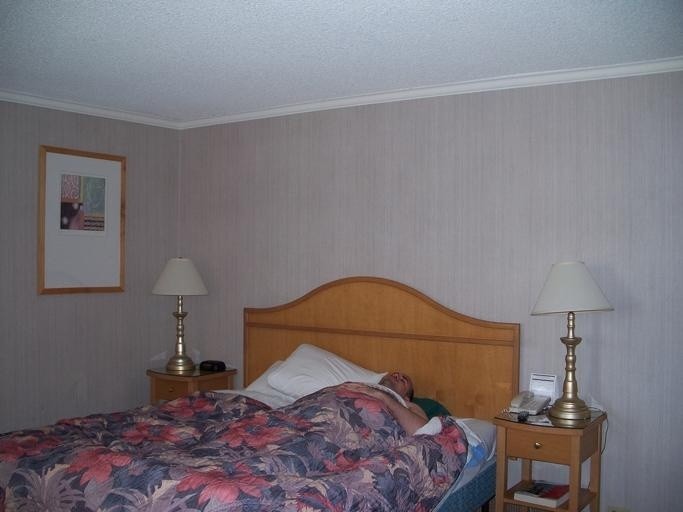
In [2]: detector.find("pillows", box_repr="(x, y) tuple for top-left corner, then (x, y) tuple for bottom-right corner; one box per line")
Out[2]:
(244, 343), (451, 421)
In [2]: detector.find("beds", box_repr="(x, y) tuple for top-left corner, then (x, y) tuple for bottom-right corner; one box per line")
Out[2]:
(1, 277), (520, 512)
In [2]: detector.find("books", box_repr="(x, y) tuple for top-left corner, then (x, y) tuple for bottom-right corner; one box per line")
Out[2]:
(514, 480), (570, 508)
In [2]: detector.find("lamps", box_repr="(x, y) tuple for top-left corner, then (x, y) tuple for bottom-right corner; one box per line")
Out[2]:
(151, 254), (210, 372)
(531, 262), (615, 421)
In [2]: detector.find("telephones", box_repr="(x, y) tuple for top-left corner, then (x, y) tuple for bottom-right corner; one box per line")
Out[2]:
(509, 392), (552, 416)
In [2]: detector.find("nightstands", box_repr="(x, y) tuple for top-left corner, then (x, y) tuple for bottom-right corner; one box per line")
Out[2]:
(493, 403), (607, 512)
(146, 367), (237, 405)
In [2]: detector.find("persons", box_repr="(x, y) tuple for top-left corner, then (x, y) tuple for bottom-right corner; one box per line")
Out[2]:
(352, 371), (430, 434)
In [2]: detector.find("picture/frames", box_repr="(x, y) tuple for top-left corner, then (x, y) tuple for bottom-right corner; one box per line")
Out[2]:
(38, 143), (127, 297)
